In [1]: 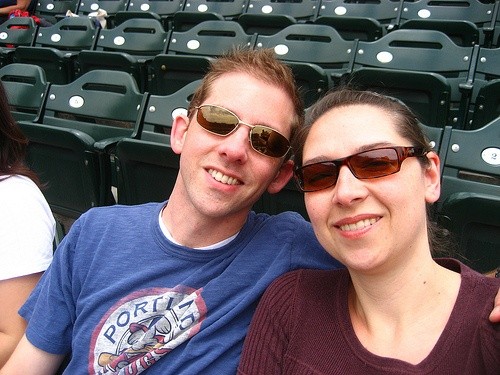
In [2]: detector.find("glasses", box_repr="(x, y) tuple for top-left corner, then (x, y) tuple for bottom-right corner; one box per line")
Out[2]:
(194, 104), (294, 158)
(293, 147), (422, 192)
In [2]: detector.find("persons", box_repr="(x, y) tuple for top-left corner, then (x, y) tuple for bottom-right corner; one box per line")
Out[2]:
(0, 47), (500, 375)
(0, 80), (56, 369)
(0, 0), (30, 25)
(237, 90), (500, 375)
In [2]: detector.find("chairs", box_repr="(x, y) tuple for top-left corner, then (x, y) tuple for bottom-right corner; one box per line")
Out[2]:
(1, 0), (500, 273)
